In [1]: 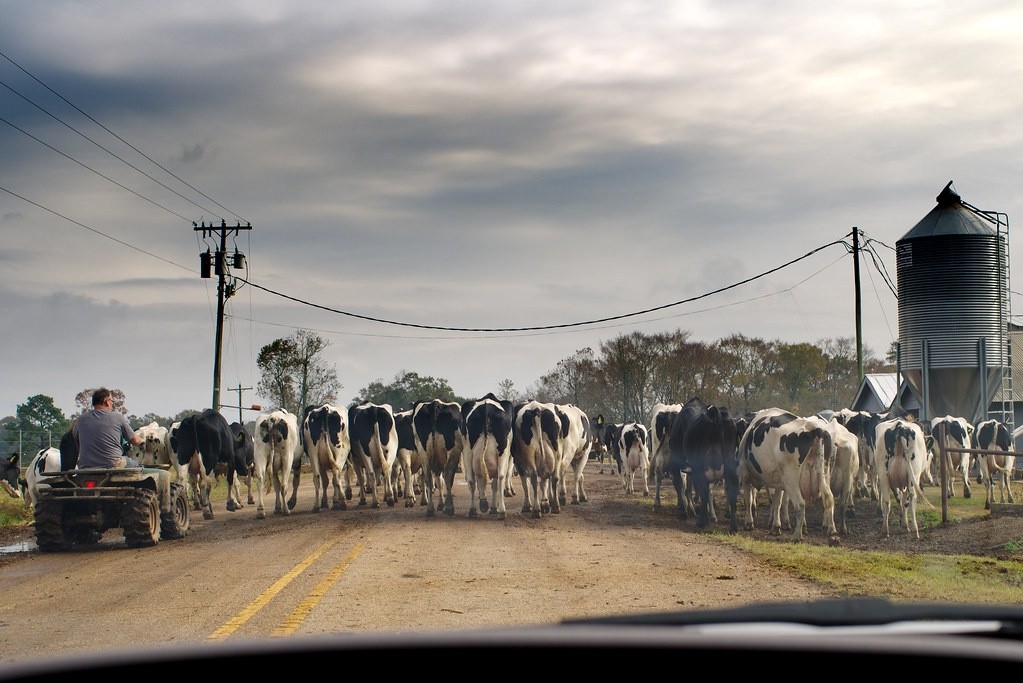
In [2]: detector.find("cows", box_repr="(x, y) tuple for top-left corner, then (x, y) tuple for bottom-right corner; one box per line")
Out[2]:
(0, 451), (22, 499)
(605, 393), (1017, 547)
(21, 392), (595, 519)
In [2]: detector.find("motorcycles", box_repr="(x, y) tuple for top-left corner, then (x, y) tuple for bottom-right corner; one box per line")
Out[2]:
(34, 439), (191, 552)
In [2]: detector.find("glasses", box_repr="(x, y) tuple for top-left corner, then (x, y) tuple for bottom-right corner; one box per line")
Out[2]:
(108, 399), (114, 404)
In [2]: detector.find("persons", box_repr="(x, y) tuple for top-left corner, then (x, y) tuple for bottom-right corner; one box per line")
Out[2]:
(70, 388), (144, 470)
